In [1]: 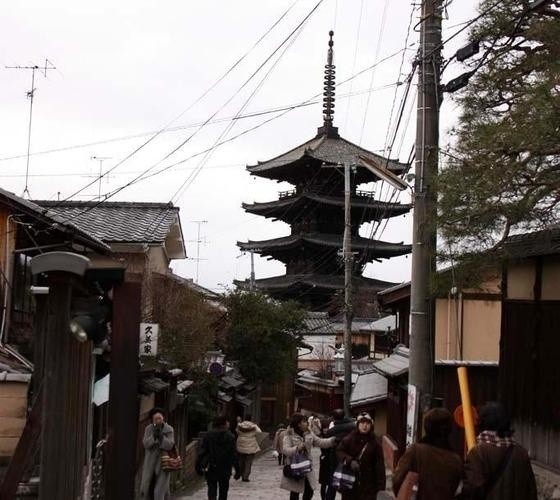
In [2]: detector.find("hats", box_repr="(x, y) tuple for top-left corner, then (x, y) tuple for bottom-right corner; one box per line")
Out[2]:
(358, 411), (373, 426)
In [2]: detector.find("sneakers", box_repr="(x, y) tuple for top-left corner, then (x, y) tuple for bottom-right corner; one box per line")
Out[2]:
(242, 476), (249, 481)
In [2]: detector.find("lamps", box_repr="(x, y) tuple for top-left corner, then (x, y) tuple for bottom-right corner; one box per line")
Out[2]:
(69, 310), (103, 344)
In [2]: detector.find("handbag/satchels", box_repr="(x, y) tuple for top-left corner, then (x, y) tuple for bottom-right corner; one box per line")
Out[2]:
(396, 444), (422, 500)
(195, 448), (210, 474)
(283, 448), (312, 477)
(332, 463), (357, 493)
(160, 450), (183, 471)
(491, 444), (530, 500)
(82, 459), (94, 500)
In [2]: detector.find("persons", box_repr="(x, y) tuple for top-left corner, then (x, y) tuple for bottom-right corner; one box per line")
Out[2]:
(390, 407), (466, 500)
(139, 407), (176, 500)
(231, 416), (243, 442)
(455, 400), (539, 500)
(235, 414), (262, 481)
(196, 415), (241, 500)
(272, 408), (386, 500)
